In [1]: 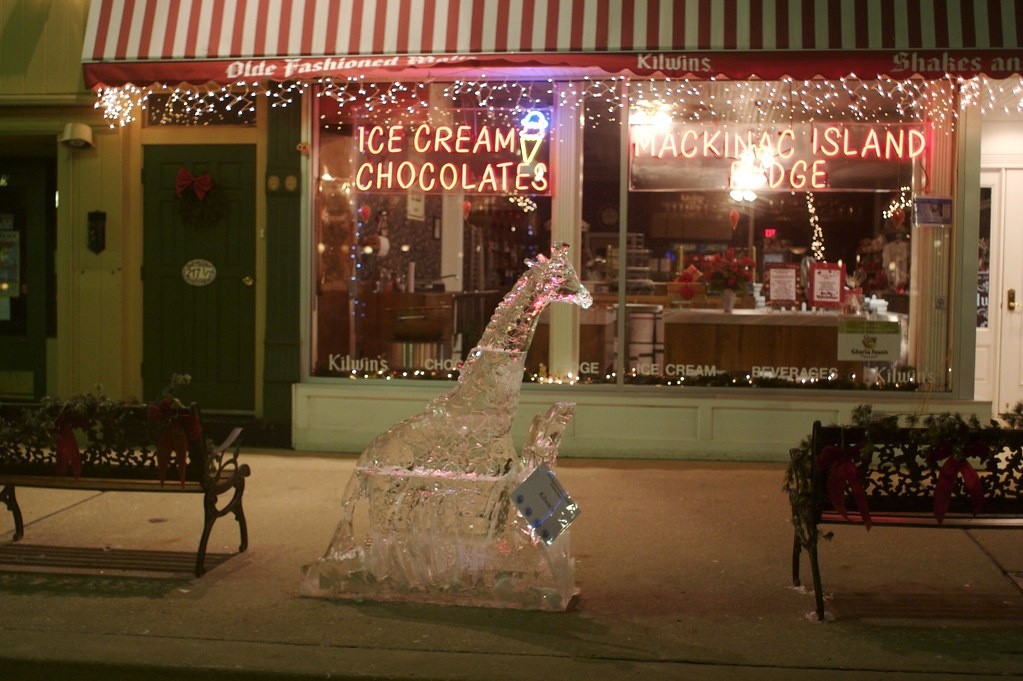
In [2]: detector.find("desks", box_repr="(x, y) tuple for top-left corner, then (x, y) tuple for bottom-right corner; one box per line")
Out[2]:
(591, 294), (865, 309)
(868, 285), (909, 315)
(316, 289), (500, 379)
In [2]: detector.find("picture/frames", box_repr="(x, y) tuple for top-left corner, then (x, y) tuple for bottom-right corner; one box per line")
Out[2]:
(433, 216), (442, 241)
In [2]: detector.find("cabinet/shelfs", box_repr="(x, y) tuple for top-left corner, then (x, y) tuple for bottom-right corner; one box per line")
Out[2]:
(762, 204), (858, 292)
(465, 206), (541, 297)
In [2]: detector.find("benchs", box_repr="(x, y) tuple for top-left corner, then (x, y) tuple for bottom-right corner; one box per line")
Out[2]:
(790, 420), (1023, 621)
(0, 394), (251, 577)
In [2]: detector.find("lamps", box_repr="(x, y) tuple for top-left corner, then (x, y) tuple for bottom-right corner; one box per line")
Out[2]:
(56, 107), (94, 150)
